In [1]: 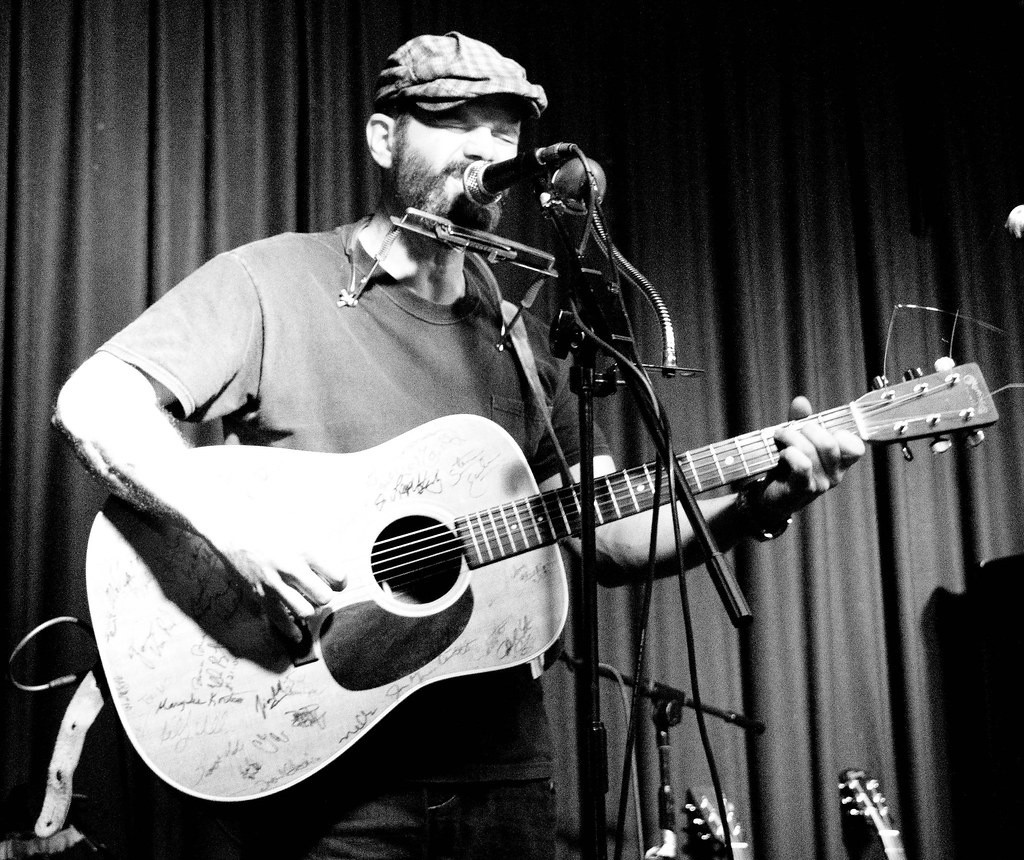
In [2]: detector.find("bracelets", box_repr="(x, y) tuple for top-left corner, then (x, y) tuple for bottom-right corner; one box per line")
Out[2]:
(736, 482), (789, 542)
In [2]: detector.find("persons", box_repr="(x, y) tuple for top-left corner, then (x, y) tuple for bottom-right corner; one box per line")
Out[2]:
(50, 30), (867, 860)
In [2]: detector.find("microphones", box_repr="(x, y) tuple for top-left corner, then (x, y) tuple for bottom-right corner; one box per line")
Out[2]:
(462, 142), (565, 210)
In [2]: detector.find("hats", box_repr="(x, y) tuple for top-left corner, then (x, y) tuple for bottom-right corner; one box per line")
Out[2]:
(370, 32), (549, 120)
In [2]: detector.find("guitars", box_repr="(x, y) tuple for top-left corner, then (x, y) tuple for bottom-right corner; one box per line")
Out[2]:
(678, 785), (757, 860)
(82, 358), (1006, 803)
(839, 766), (912, 860)
